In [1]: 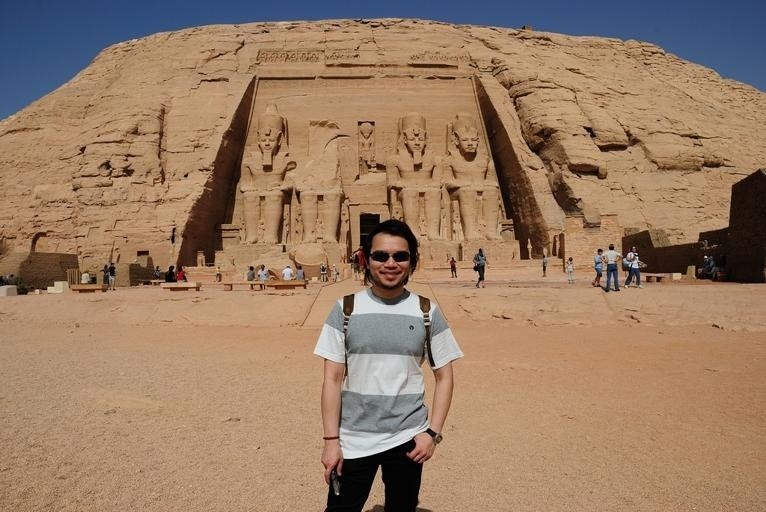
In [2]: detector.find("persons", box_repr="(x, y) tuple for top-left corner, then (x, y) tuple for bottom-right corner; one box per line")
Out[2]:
(359, 123), (375, 174)
(443, 117), (507, 240)
(386, 115), (443, 240)
(473, 248), (490, 288)
(450, 257), (458, 278)
(593, 244), (643, 292)
(241, 114), (295, 244)
(566, 257), (575, 284)
(542, 256), (547, 277)
(81, 245), (369, 292)
(313, 220), (465, 512)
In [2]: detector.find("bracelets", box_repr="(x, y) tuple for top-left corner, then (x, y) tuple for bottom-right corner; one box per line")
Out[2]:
(487, 265), (490, 266)
(323, 436), (339, 440)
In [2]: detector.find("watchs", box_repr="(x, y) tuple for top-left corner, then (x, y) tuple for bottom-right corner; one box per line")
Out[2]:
(426, 428), (443, 444)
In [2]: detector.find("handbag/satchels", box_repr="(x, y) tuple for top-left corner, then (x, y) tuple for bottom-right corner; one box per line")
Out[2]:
(623, 258), (632, 268)
(473, 266), (478, 271)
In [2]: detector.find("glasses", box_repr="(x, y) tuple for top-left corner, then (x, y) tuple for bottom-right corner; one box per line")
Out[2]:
(369, 250), (413, 262)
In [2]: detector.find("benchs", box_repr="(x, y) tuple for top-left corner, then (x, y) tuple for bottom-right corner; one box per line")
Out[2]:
(70, 278), (307, 294)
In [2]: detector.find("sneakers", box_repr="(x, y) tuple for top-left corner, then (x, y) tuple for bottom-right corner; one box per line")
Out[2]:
(597, 284), (602, 287)
(476, 284), (480, 288)
(624, 285), (628, 288)
(637, 285), (641, 288)
(592, 282), (596, 286)
(605, 290), (609, 292)
(615, 289), (620, 291)
